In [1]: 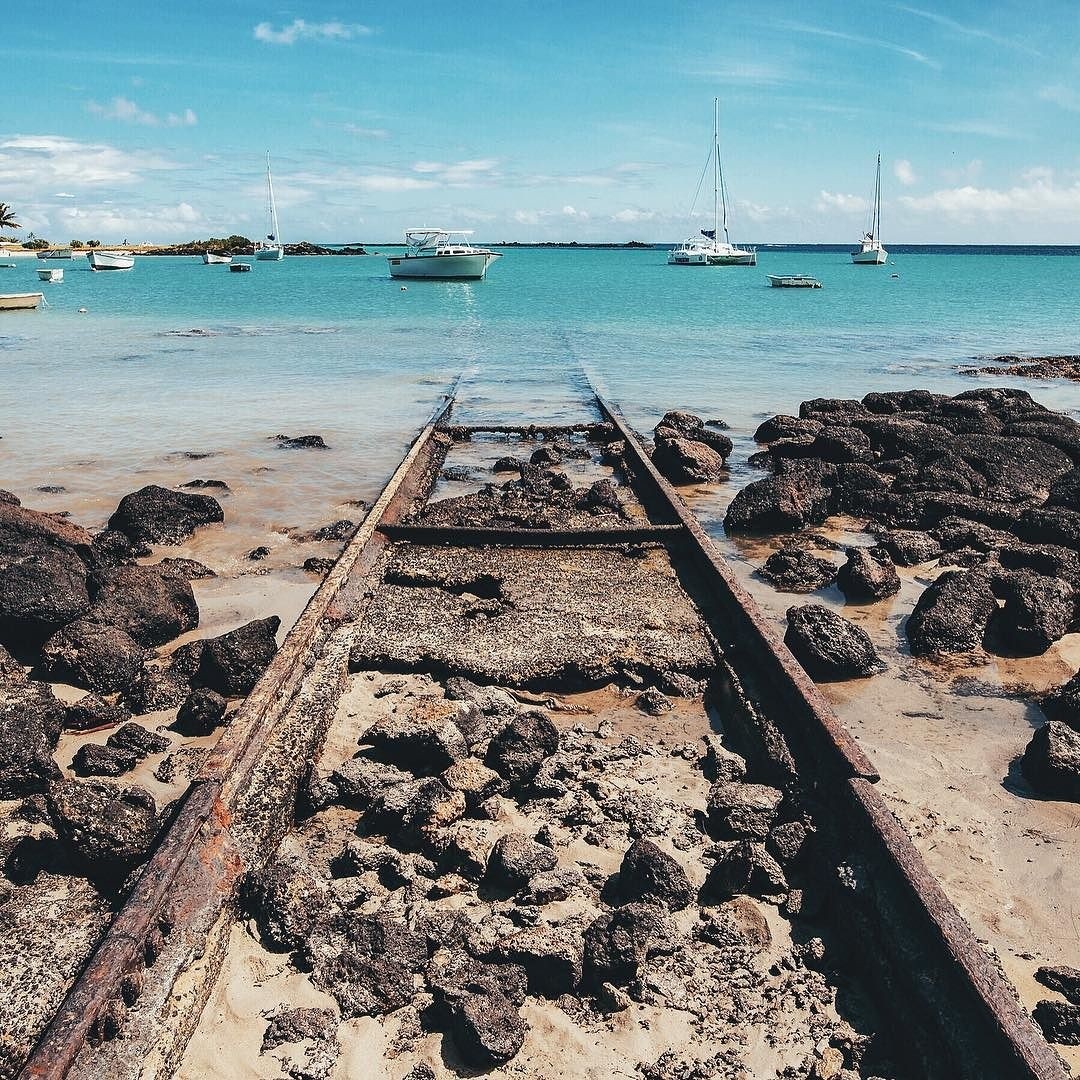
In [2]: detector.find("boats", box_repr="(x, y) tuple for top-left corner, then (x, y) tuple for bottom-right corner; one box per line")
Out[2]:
(201, 249), (234, 265)
(385, 226), (504, 279)
(85, 249), (136, 270)
(765, 273), (821, 289)
(36, 247), (73, 259)
(666, 245), (712, 265)
(36, 268), (64, 283)
(0, 293), (44, 310)
(230, 262), (252, 273)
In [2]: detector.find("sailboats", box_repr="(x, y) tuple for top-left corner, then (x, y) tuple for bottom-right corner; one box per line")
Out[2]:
(688, 96), (758, 266)
(850, 149), (890, 266)
(252, 148), (284, 261)
